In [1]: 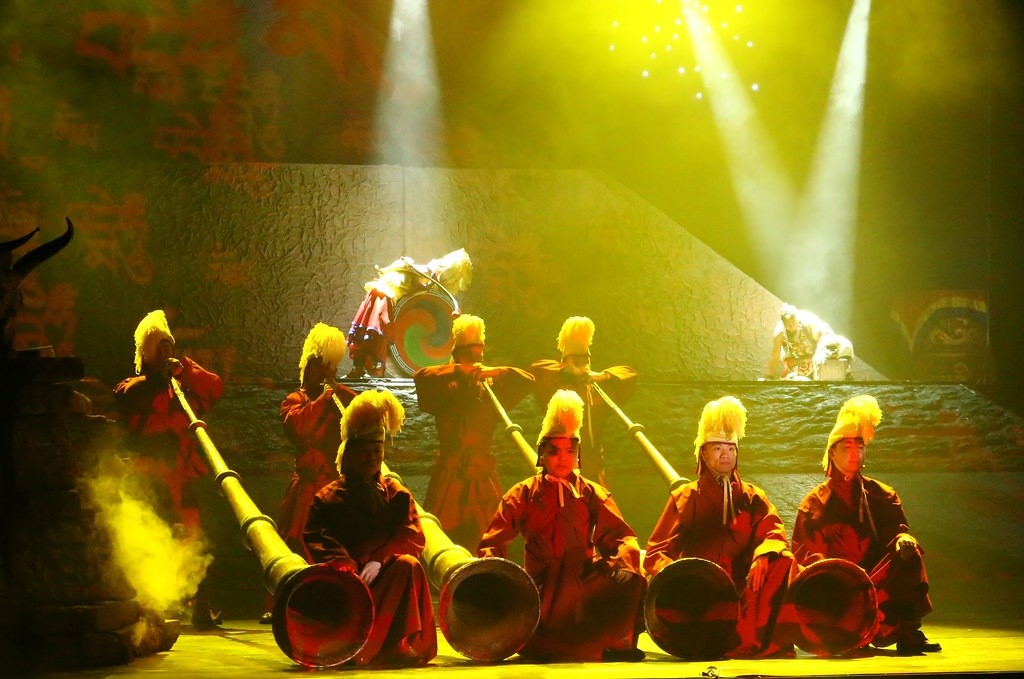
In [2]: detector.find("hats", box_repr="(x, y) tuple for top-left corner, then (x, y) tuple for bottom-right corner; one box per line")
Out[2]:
(427, 248), (473, 297)
(693, 396), (747, 475)
(299, 323), (346, 390)
(821, 395), (883, 478)
(536, 390), (585, 469)
(134, 310), (175, 374)
(557, 316), (596, 364)
(451, 314), (486, 363)
(335, 389), (407, 477)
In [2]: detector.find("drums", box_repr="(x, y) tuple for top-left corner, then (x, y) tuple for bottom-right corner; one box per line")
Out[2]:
(384, 288), (461, 379)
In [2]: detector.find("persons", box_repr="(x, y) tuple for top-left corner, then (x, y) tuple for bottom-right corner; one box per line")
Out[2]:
(531, 314), (638, 489)
(644, 395), (797, 658)
(415, 313), (536, 561)
(788, 395), (943, 653)
(259, 321), (360, 625)
(111, 308), (225, 633)
(476, 388), (650, 662)
(337, 246), (474, 383)
(298, 385), (439, 672)
(762, 304), (855, 381)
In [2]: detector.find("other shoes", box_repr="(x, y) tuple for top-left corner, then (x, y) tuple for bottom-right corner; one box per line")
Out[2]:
(259, 605), (275, 623)
(387, 646), (427, 664)
(602, 647), (645, 663)
(897, 631), (941, 653)
(192, 605), (223, 628)
(345, 361), (384, 377)
